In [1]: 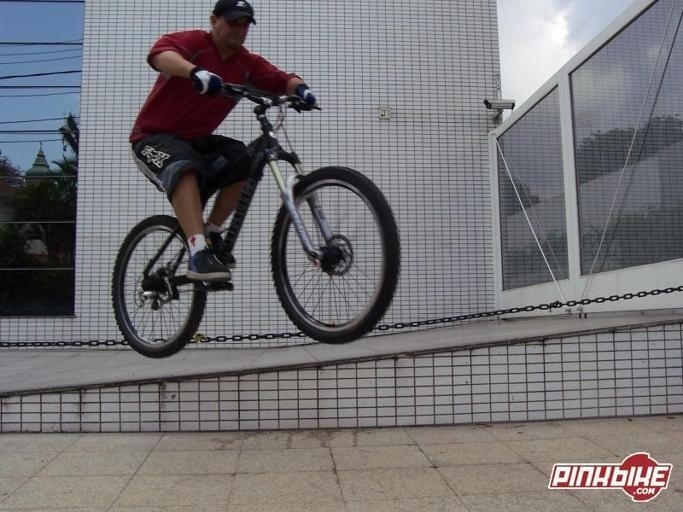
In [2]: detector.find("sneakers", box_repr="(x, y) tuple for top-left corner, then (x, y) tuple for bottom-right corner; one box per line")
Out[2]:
(185, 231), (236, 280)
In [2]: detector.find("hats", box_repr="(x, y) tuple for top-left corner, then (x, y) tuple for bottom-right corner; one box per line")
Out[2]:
(212, 1), (257, 26)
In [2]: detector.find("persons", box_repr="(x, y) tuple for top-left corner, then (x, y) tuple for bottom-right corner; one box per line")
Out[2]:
(128, 0), (316, 282)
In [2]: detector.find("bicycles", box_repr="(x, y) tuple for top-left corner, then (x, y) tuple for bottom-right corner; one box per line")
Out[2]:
(106, 73), (405, 361)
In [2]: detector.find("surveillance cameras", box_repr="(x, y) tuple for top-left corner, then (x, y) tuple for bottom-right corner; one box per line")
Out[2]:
(484, 99), (516, 109)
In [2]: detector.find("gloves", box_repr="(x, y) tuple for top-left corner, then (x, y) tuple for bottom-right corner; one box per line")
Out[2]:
(190, 67), (224, 97)
(293, 85), (316, 110)
